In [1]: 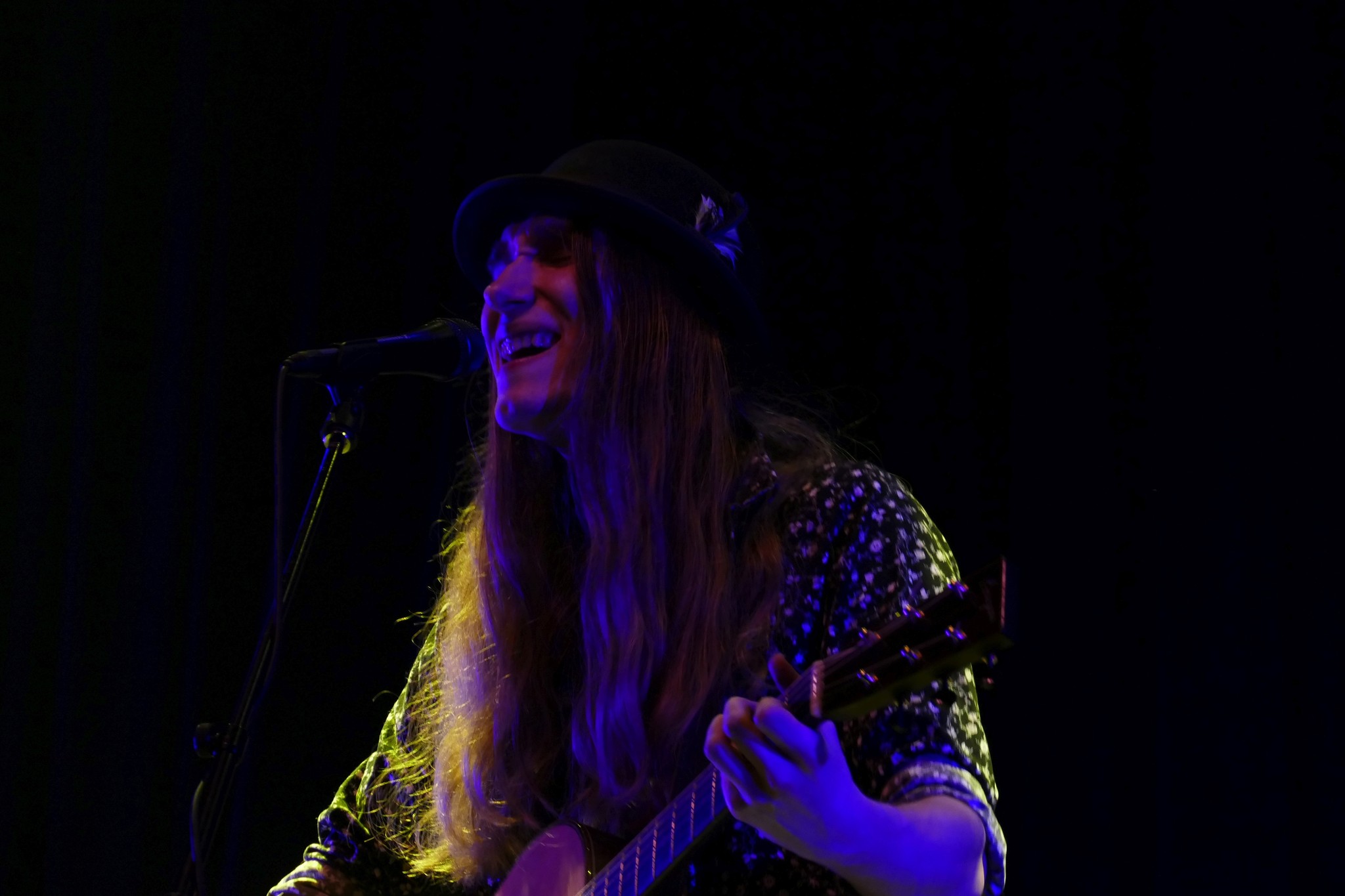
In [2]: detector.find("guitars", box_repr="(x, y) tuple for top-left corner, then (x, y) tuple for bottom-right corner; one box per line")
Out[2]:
(487, 554), (1019, 895)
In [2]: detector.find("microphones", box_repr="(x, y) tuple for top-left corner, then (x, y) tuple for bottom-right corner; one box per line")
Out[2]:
(288, 318), (488, 382)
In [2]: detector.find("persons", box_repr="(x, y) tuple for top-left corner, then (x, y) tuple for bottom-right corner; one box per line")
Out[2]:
(266, 141), (1009, 896)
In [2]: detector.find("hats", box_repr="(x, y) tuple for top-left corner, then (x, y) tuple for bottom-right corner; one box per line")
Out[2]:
(453, 139), (768, 385)
